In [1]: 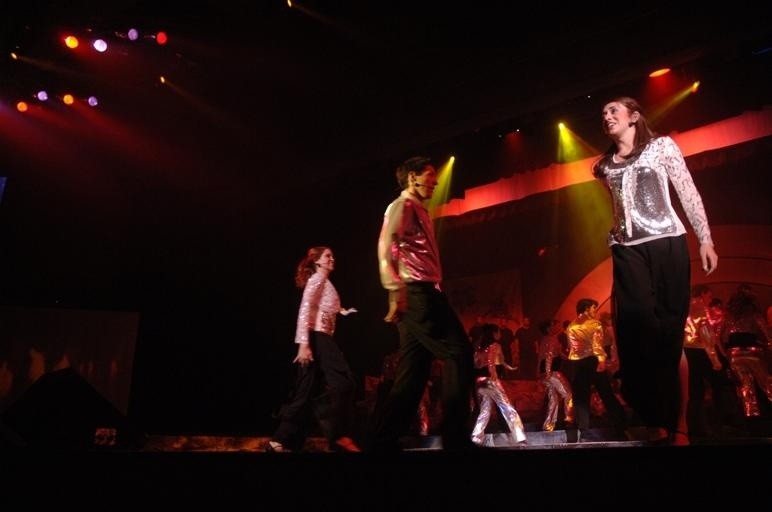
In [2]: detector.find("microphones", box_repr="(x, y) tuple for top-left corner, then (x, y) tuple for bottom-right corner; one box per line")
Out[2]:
(414, 182), (421, 187)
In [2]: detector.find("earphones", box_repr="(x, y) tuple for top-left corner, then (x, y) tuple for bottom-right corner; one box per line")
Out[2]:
(410, 174), (414, 178)
(315, 262), (321, 267)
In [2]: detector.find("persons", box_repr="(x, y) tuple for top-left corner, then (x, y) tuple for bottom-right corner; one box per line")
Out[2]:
(591, 94), (720, 445)
(264, 243), (364, 454)
(379, 281), (772, 446)
(372, 155), (489, 448)
